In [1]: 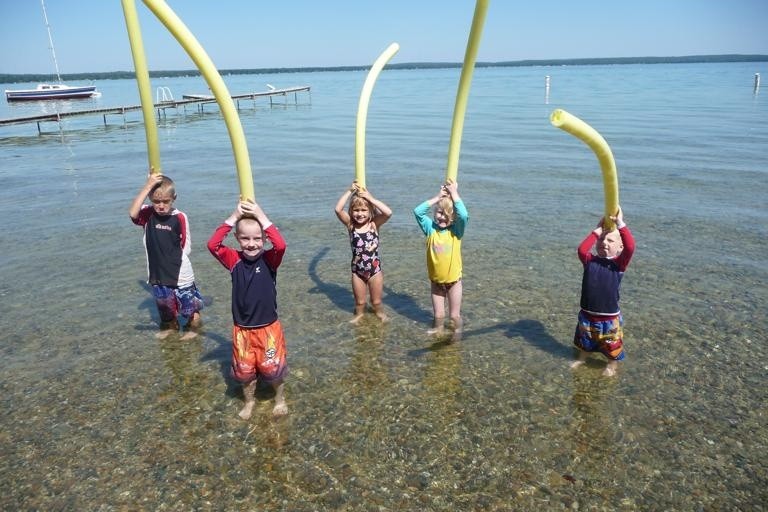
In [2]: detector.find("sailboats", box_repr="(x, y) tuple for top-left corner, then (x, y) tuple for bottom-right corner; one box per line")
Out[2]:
(5, 0), (97, 103)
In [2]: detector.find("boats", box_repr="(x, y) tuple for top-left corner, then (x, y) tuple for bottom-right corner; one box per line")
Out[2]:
(182, 94), (217, 100)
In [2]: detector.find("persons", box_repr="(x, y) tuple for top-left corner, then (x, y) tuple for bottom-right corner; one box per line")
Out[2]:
(207, 193), (290, 419)
(413, 179), (470, 337)
(128, 166), (203, 341)
(334, 179), (393, 324)
(568, 206), (636, 378)
